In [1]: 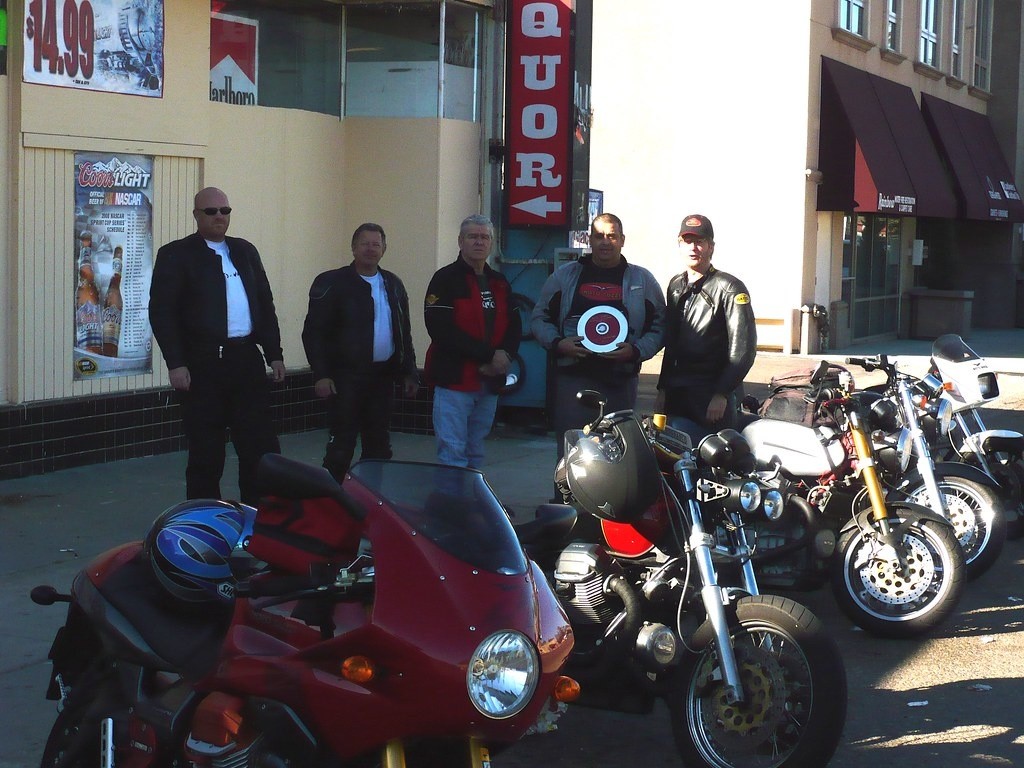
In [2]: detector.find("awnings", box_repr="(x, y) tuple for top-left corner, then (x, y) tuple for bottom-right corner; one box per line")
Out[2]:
(817, 55), (1024, 223)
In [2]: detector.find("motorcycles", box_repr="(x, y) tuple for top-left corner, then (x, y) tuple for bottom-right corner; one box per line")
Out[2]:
(30, 451), (587, 768)
(408, 390), (852, 768)
(643, 333), (1024, 636)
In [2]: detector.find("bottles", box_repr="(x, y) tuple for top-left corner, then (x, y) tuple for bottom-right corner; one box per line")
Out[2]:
(103, 246), (123, 358)
(75, 230), (104, 354)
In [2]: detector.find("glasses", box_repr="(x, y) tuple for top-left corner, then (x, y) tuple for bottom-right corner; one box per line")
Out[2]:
(679, 237), (713, 245)
(197, 207), (232, 215)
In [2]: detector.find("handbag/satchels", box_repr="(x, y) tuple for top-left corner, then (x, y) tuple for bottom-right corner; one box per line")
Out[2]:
(757, 363), (855, 429)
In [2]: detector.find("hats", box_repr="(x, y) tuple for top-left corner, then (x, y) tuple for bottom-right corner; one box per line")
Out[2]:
(678, 214), (714, 239)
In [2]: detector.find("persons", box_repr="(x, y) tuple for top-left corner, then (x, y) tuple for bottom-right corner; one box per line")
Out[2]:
(531, 213), (665, 503)
(301, 223), (420, 484)
(422, 215), (522, 521)
(655, 215), (757, 434)
(148, 187), (286, 504)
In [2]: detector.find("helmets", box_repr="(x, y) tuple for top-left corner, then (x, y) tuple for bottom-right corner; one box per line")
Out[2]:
(143, 498), (271, 602)
(563, 408), (661, 523)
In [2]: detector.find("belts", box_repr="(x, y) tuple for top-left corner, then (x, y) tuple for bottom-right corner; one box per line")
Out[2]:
(227, 334), (256, 347)
